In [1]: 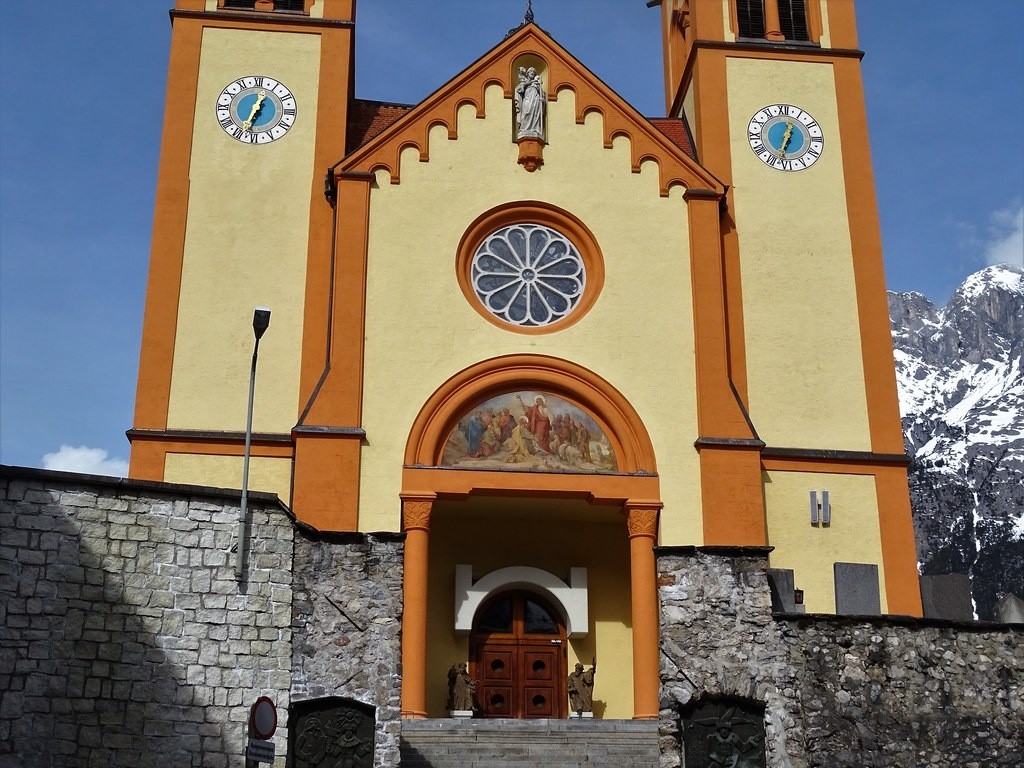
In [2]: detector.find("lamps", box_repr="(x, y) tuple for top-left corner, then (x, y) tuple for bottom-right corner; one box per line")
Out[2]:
(232, 305), (272, 582)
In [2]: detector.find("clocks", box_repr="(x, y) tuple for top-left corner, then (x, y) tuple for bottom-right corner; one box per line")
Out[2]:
(216, 75), (297, 146)
(747, 103), (824, 172)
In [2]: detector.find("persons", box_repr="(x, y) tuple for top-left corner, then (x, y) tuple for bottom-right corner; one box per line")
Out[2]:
(513, 66), (547, 143)
(447, 662), (478, 712)
(566, 663), (596, 715)
